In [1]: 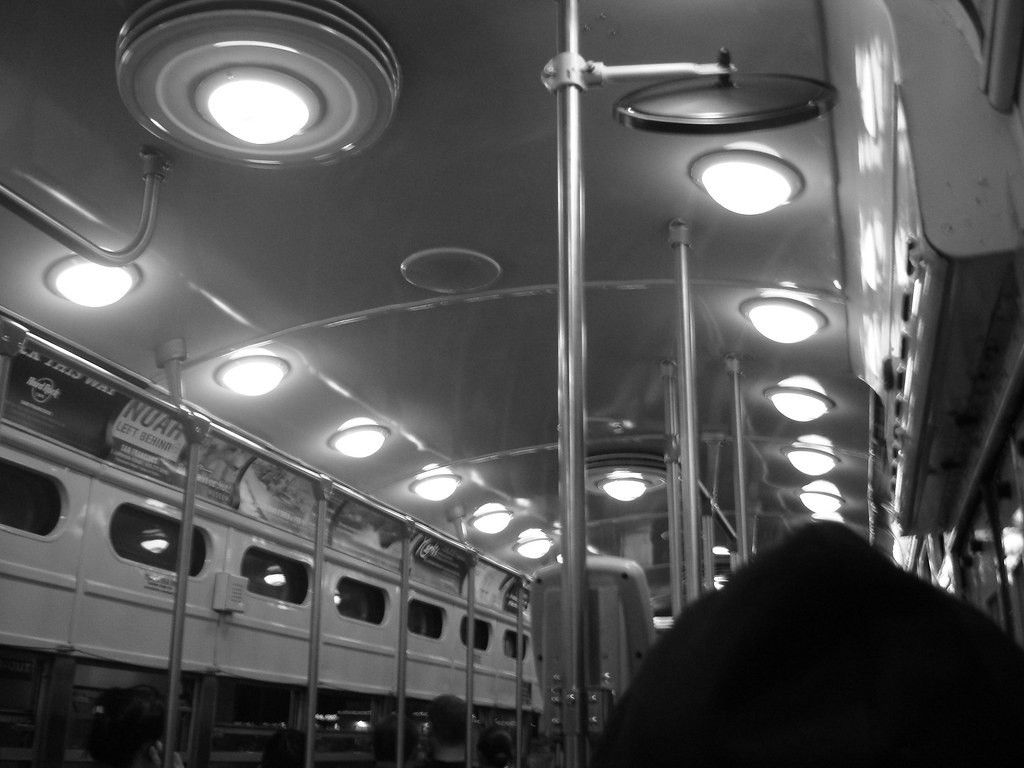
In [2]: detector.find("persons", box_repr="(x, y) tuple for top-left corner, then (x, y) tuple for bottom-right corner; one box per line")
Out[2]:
(586, 521), (1024, 768)
(259, 728), (306, 768)
(477, 727), (515, 768)
(420, 693), (476, 768)
(371, 714), (420, 768)
(83, 684), (184, 768)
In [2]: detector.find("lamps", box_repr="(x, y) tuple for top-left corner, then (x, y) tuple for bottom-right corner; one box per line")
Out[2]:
(197, 66), (322, 148)
(596, 474), (653, 502)
(779, 446), (840, 476)
(214, 355), (288, 397)
(510, 536), (554, 559)
(45, 255), (139, 309)
(467, 510), (514, 534)
(793, 489), (844, 513)
(739, 296), (827, 345)
(408, 473), (462, 501)
(690, 150), (804, 218)
(327, 425), (389, 458)
(762, 386), (834, 422)
